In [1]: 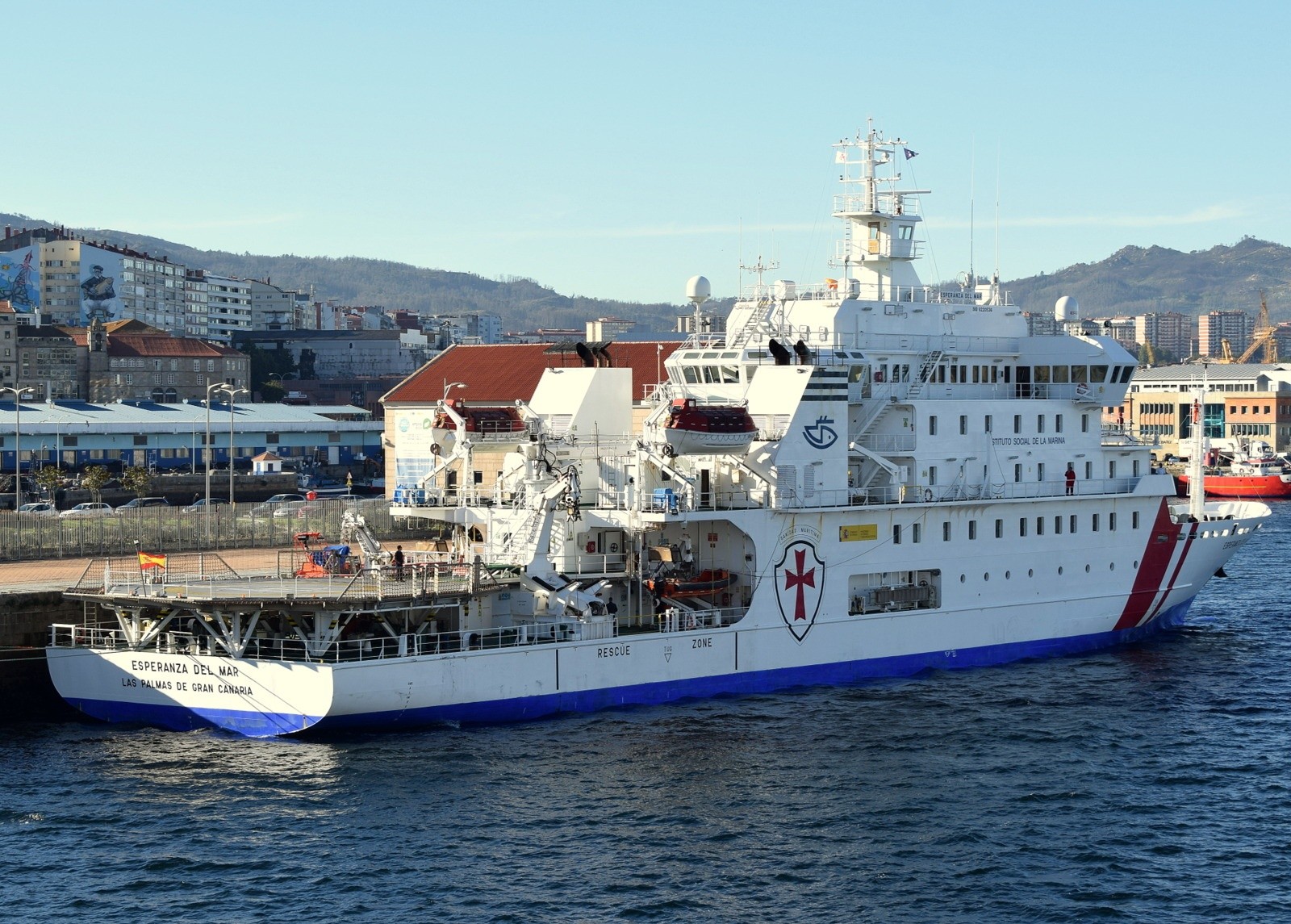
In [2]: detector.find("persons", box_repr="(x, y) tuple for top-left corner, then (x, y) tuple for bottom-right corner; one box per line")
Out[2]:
(1187, 514), (1198, 523)
(1065, 467), (1075, 495)
(1155, 463), (1166, 474)
(676, 533), (694, 580)
(191, 610), (286, 651)
(653, 597), (663, 622)
(394, 545), (405, 582)
(894, 194), (903, 215)
(1191, 398), (1201, 424)
(606, 597), (618, 633)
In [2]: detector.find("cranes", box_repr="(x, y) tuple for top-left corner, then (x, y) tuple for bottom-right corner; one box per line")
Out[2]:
(1190, 336), (1239, 365)
(1235, 292), (1284, 364)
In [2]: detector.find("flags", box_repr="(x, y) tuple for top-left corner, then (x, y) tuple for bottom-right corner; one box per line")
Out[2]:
(835, 150), (847, 164)
(137, 552), (166, 569)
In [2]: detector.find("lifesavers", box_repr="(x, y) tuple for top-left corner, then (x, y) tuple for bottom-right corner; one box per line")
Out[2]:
(1024, 311), (1030, 317)
(1249, 468), (1254, 474)
(924, 488), (933, 500)
(1077, 383), (1088, 394)
(1239, 468), (1244, 473)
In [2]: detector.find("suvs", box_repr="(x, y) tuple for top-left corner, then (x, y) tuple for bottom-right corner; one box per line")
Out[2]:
(114, 497), (171, 516)
(249, 494), (307, 518)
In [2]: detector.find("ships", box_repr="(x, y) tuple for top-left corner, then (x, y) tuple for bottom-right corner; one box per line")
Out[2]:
(35, 114), (1274, 738)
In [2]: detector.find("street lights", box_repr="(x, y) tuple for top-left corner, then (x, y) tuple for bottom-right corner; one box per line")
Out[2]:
(57, 415), (70, 469)
(0, 386), (35, 558)
(213, 387), (250, 548)
(206, 382), (233, 547)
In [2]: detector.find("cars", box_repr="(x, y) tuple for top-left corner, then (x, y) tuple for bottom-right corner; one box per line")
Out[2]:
(337, 494), (386, 519)
(298, 498), (344, 519)
(273, 501), (307, 518)
(58, 502), (115, 520)
(12, 502), (56, 521)
(182, 497), (233, 516)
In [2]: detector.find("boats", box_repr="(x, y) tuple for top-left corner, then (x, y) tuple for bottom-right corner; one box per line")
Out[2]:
(641, 567), (739, 597)
(1179, 423), (1291, 499)
(661, 397), (760, 456)
